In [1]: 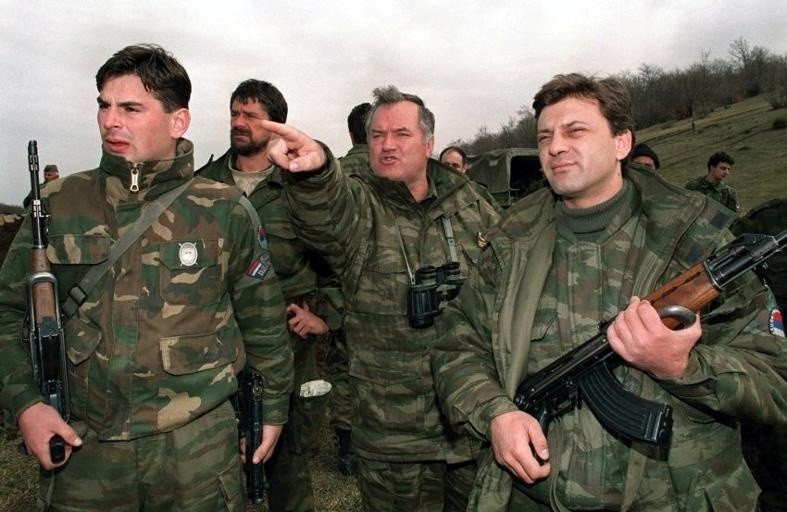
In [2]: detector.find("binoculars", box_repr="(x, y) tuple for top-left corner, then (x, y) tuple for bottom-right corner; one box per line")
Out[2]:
(407, 259), (469, 328)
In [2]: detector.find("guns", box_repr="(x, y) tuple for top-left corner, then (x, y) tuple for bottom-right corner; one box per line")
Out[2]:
(231, 366), (264, 506)
(512, 231), (787, 460)
(27, 139), (71, 463)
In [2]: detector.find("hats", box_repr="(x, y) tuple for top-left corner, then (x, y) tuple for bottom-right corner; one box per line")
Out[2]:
(631, 143), (659, 169)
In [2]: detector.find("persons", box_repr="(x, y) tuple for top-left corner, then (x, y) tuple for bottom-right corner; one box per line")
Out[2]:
(260, 83), (500, 512)
(435, 72), (787, 512)
(522, 168), (548, 198)
(685, 152), (738, 211)
(326, 103), (372, 479)
(440, 147), (503, 218)
(633, 142), (661, 172)
(23, 164), (60, 208)
(199, 75), (343, 512)
(0, 42), (294, 512)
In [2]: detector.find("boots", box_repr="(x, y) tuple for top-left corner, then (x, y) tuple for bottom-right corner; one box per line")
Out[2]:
(337, 429), (354, 476)
